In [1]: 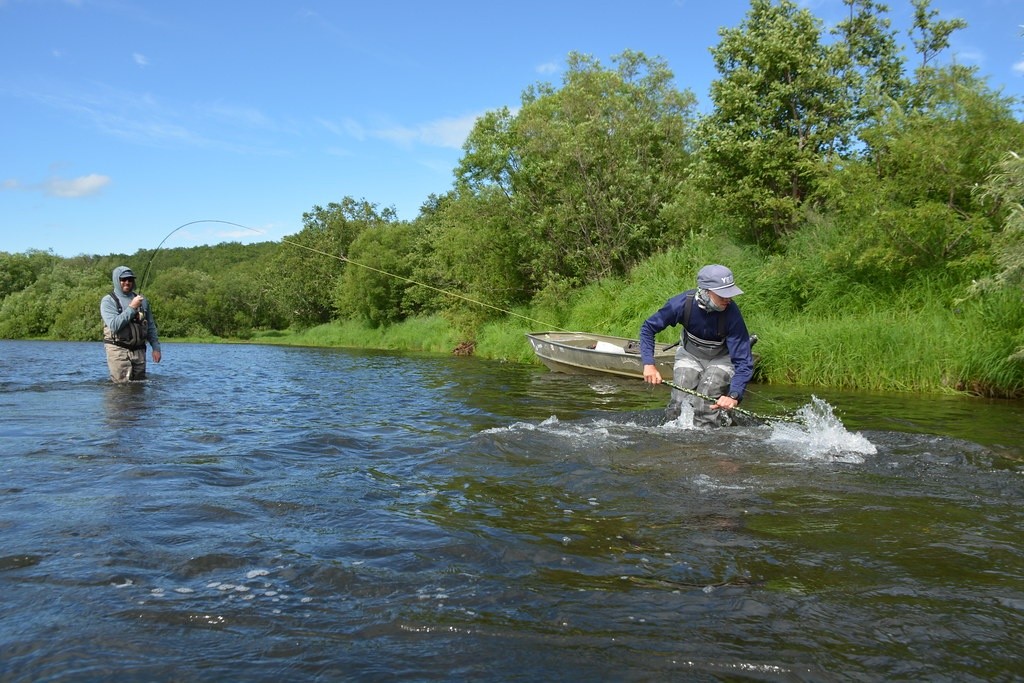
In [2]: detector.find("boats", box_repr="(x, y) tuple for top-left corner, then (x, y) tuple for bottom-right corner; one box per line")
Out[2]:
(525, 329), (760, 384)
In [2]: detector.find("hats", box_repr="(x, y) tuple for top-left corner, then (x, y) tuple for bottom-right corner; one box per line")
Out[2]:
(119, 270), (137, 279)
(696, 264), (745, 298)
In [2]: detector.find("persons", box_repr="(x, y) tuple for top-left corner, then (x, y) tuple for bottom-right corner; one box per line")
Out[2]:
(100, 265), (161, 382)
(639, 264), (754, 427)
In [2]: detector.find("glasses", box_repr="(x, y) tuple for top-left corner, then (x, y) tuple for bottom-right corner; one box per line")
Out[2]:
(119, 278), (134, 282)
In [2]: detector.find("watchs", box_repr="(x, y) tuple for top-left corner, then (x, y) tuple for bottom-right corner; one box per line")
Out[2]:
(727, 391), (741, 403)
